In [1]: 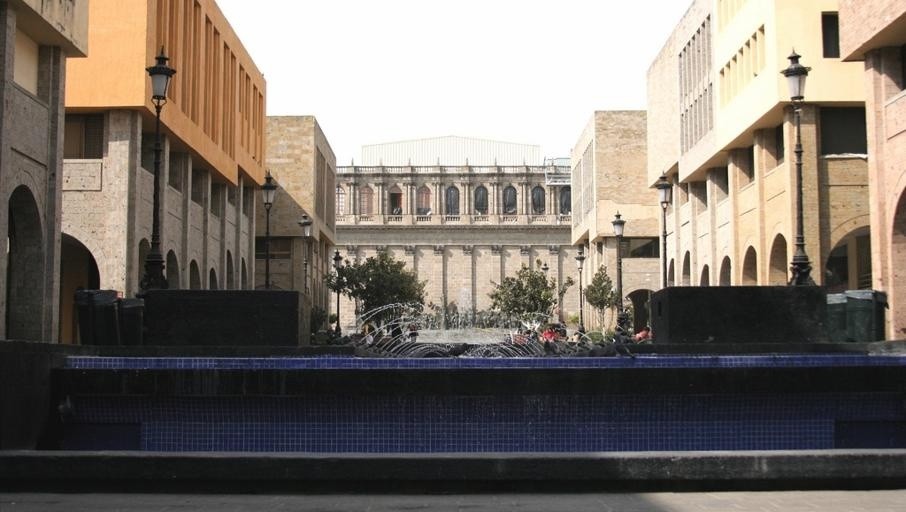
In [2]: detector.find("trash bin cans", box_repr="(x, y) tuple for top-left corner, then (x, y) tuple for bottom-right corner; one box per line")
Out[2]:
(823, 289), (889, 341)
(74, 288), (145, 345)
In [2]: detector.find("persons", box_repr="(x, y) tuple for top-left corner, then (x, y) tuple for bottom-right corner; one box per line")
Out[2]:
(504, 320), (589, 343)
(634, 326), (653, 344)
(390, 319), (419, 342)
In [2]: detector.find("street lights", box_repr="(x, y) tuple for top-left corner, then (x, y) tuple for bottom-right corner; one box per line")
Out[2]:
(263, 173), (277, 290)
(301, 217), (313, 293)
(142, 47), (176, 289)
(781, 53), (816, 285)
(613, 213), (625, 315)
(656, 173), (674, 289)
(576, 252), (589, 331)
(334, 251), (345, 338)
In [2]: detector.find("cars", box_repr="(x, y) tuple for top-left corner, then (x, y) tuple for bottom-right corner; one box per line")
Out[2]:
(543, 323), (567, 337)
